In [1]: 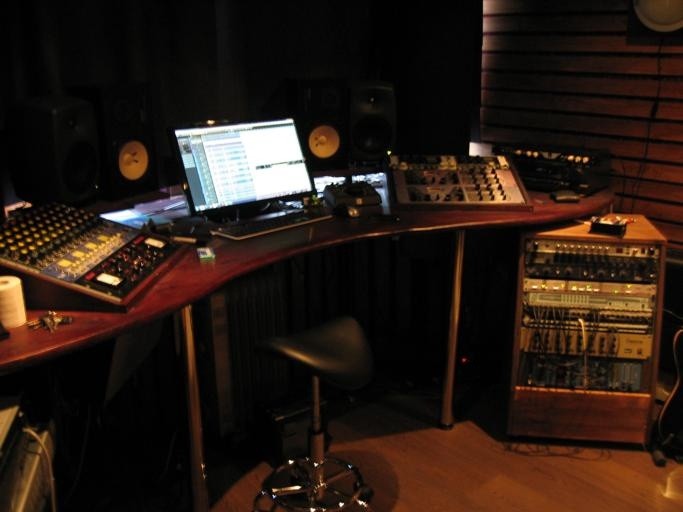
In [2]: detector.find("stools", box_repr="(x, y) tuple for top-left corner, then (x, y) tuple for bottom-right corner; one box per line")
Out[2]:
(264, 315), (374, 511)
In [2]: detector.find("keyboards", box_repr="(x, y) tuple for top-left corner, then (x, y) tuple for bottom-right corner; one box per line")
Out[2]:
(212, 207), (331, 241)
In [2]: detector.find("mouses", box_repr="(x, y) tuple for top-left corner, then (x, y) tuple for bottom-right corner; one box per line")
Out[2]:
(339, 203), (360, 217)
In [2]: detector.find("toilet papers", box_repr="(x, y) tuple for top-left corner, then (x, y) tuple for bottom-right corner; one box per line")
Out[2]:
(0, 275), (27, 330)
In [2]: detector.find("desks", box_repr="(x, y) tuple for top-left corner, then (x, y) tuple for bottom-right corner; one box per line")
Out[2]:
(1, 184), (618, 512)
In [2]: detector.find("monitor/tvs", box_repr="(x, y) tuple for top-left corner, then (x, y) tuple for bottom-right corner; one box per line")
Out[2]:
(171, 114), (317, 222)
